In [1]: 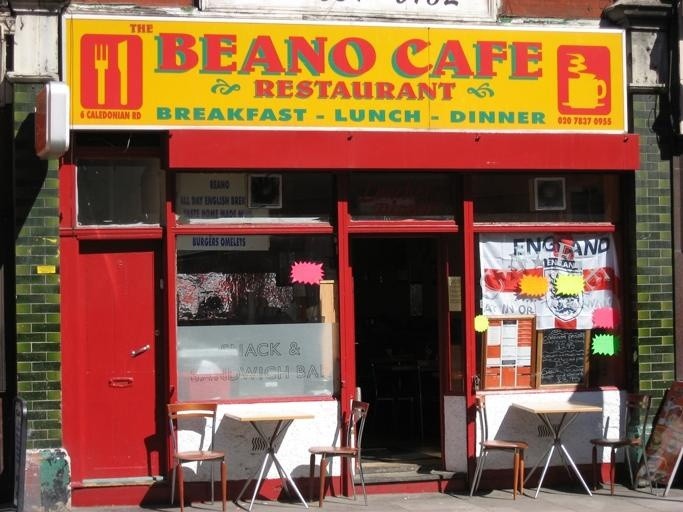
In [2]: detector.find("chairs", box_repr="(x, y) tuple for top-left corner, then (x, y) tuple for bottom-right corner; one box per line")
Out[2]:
(167, 404), (226, 512)
(468, 395), (528, 499)
(590, 394), (655, 496)
(308, 401), (369, 508)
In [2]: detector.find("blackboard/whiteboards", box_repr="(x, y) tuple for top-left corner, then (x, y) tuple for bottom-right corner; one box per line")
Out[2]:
(535, 328), (591, 389)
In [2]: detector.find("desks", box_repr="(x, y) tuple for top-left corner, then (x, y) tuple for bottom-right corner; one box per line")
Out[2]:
(513, 400), (603, 500)
(224, 413), (315, 512)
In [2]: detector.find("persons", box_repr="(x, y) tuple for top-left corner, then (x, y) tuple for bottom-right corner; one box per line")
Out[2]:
(249, 295), (292, 323)
(194, 297), (223, 321)
